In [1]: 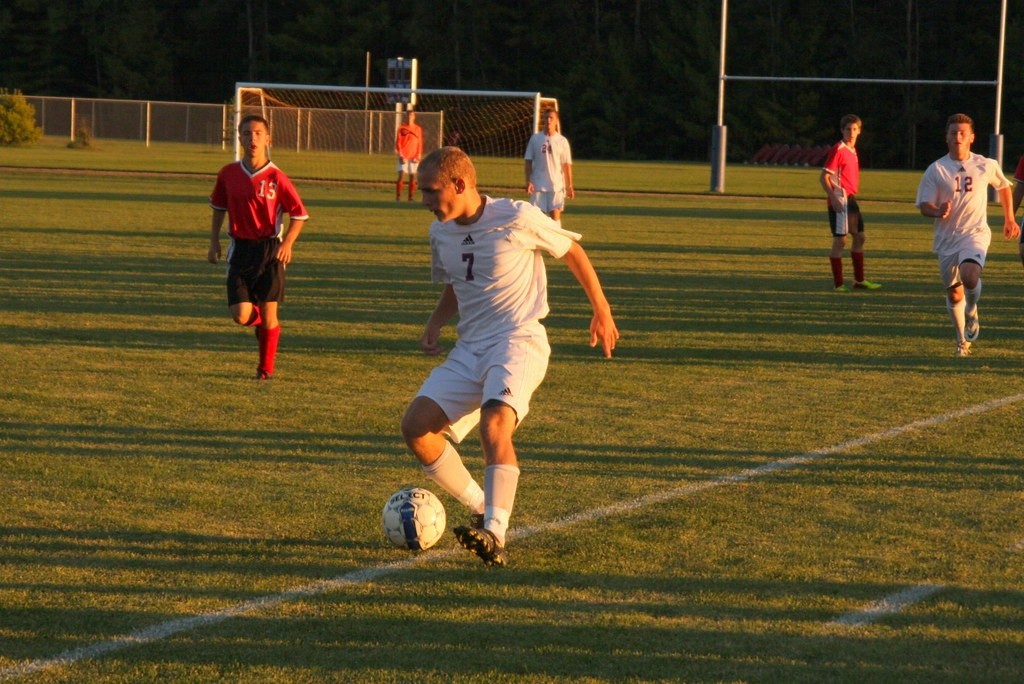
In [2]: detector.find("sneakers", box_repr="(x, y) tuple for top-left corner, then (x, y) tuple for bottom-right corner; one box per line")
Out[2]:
(453, 526), (508, 567)
(833, 284), (849, 292)
(958, 340), (971, 358)
(964, 304), (981, 342)
(470, 514), (484, 529)
(852, 280), (881, 289)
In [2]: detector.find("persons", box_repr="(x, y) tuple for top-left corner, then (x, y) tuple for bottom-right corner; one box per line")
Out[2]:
(819, 114), (882, 291)
(524, 109), (575, 228)
(207, 116), (309, 380)
(396, 110), (423, 209)
(916, 114), (1024, 355)
(401, 146), (619, 568)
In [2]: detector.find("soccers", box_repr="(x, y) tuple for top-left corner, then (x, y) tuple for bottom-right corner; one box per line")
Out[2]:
(381, 486), (448, 551)
